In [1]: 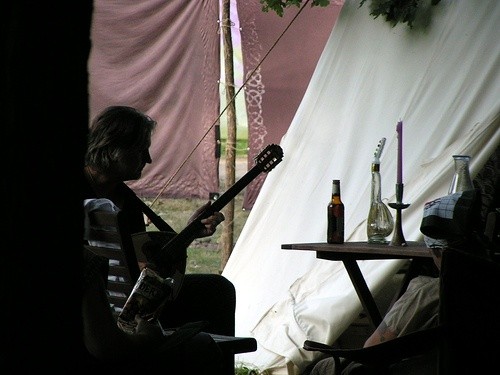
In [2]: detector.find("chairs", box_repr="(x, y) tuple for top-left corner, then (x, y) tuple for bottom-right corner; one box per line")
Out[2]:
(304, 190), (500, 375)
(83, 199), (257, 375)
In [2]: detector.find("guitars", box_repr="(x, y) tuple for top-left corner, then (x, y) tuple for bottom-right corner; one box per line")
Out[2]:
(129, 144), (283, 297)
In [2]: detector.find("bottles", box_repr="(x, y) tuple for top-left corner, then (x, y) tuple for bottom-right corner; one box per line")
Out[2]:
(328, 179), (344, 244)
(367, 138), (394, 242)
(447, 155), (473, 194)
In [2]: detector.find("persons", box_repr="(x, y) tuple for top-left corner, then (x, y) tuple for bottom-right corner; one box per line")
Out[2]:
(86, 105), (236, 375)
(311, 190), (467, 375)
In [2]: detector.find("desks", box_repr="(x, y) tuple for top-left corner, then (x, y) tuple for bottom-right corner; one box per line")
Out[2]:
(281, 242), (433, 375)
